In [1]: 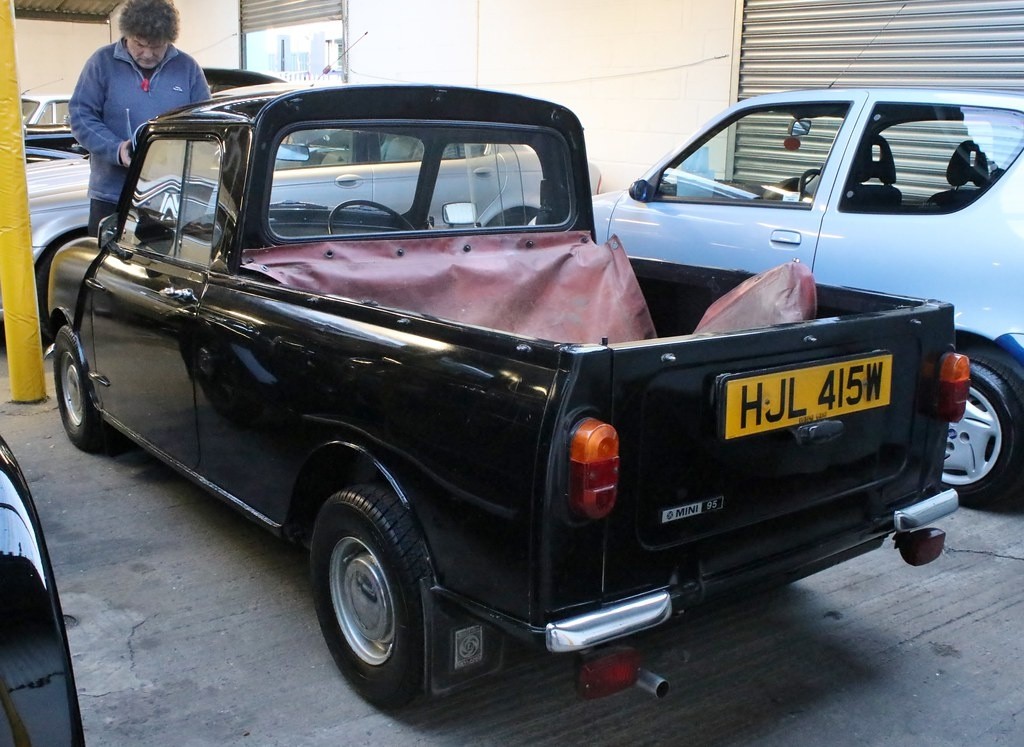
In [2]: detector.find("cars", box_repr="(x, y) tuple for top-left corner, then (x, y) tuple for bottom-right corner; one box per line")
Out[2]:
(528, 91), (1024, 505)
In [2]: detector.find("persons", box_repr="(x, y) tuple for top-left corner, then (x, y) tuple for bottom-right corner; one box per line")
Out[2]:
(68, 1), (216, 224)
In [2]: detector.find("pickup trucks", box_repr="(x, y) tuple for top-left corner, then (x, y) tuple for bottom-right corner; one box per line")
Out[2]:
(0, 69), (971, 711)
